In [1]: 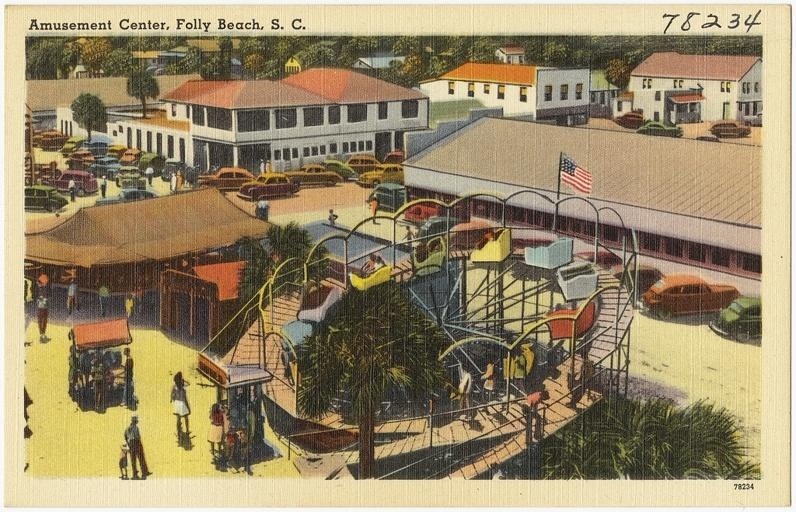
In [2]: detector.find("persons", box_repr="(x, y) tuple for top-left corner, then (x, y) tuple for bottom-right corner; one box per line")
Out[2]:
(143, 168), (156, 185)
(67, 176), (77, 202)
(25, 258), (294, 477)
(168, 172), (176, 192)
(327, 208), (339, 227)
(367, 195), (380, 225)
(100, 174), (109, 201)
(458, 364), (475, 419)
(176, 170), (182, 192)
(521, 391), (553, 446)
(403, 224), (417, 246)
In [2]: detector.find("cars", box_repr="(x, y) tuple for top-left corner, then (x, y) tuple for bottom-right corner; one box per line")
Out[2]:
(366, 183), (762, 343)
(24, 119), (185, 212)
(197, 151), (404, 201)
(613, 112), (683, 137)
(696, 112), (763, 143)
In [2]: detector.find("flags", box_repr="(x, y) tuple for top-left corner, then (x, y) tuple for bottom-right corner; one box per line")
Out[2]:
(559, 154), (592, 197)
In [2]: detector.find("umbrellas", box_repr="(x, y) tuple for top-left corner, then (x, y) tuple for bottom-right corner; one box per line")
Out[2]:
(142, 166), (156, 175)
